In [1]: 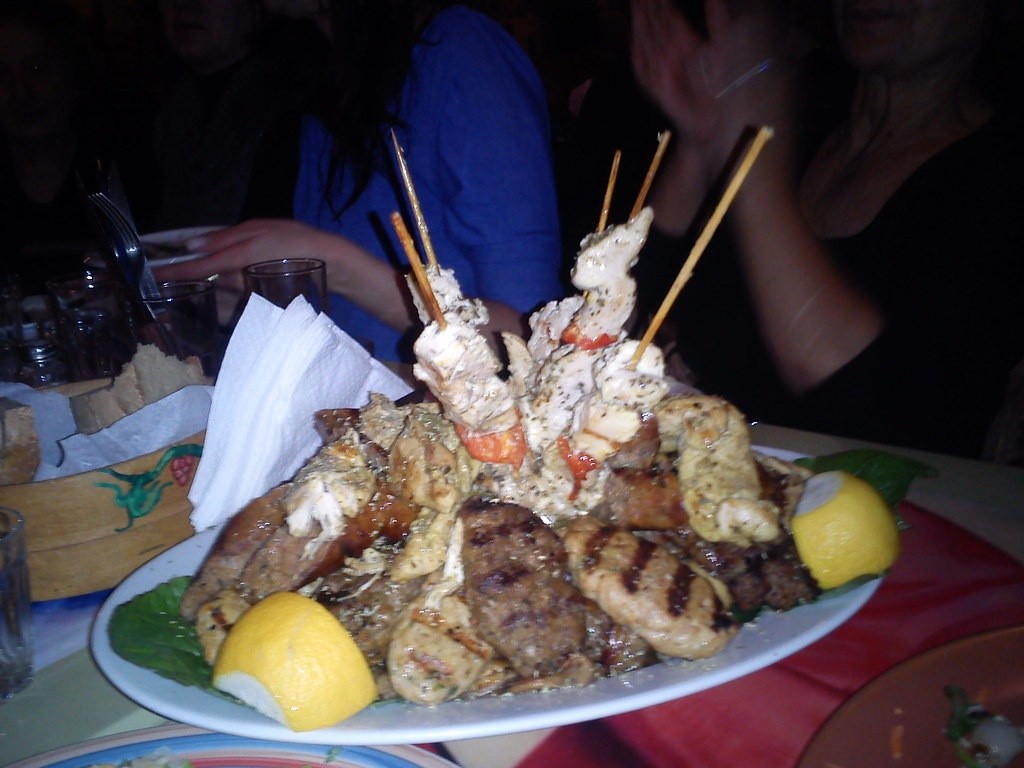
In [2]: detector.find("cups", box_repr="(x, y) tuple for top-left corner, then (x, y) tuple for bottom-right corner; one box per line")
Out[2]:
(137, 281), (218, 378)
(0, 506), (34, 698)
(0, 271), (22, 382)
(41, 271), (136, 379)
(242, 257), (326, 315)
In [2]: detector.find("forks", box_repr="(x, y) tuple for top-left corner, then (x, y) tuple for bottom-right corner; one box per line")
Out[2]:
(87, 160), (182, 359)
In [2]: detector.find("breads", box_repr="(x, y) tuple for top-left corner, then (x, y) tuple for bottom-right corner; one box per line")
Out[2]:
(0, 343), (211, 493)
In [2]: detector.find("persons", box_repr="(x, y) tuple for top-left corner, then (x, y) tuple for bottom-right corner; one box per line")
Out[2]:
(0, 1), (565, 375)
(628, 0), (1022, 466)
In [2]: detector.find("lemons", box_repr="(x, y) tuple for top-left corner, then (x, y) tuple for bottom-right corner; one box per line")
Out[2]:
(792, 467), (899, 589)
(212, 591), (379, 731)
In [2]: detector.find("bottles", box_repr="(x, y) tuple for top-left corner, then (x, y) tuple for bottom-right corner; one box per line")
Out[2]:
(16, 342), (67, 388)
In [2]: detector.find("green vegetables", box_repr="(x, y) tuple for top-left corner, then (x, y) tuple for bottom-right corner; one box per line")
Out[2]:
(109, 574), (213, 690)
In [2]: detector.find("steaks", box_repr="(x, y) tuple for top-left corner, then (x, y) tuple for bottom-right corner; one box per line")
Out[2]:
(314, 458), (808, 702)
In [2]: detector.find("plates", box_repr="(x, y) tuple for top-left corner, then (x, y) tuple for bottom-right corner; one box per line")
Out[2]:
(91, 445), (875, 747)
(84, 226), (229, 267)
(796, 625), (1024, 768)
(0, 725), (456, 768)
